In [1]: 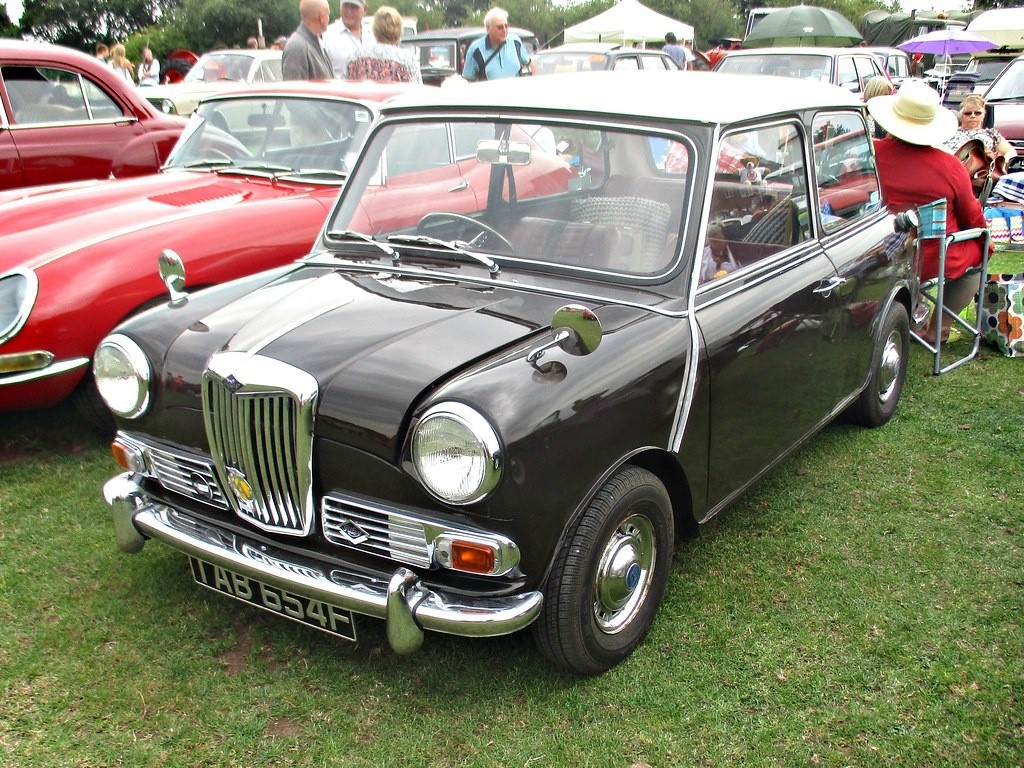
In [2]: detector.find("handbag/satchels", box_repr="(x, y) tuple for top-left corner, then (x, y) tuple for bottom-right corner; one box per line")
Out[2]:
(983, 206), (1024, 244)
(953, 139), (1006, 205)
(974, 273), (1024, 358)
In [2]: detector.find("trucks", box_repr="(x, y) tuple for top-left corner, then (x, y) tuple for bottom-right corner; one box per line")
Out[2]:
(860, 9), (971, 74)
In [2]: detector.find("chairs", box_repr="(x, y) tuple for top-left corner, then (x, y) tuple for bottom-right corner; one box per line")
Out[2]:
(903, 197), (989, 377)
(772, 273), (861, 347)
(503, 212), (613, 273)
(660, 235), (791, 285)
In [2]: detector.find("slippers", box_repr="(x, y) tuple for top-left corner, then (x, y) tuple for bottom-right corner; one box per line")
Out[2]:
(925, 325), (962, 343)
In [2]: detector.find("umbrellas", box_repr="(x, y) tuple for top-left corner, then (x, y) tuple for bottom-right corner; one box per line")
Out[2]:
(897, 29), (1000, 107)
(741, 2), (864, 48)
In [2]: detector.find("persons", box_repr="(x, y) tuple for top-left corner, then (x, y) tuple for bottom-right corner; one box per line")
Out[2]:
(462, 6), (537, 83)
(661, 33), (695, 71)
(944, 95), (1017, 199)
(632, 42), (642, 50)
(282, 0), (424, 146)
(933, 53), (952, 68)
(204, 37), (288, 84)
(138, 46), (160, 87)
(96, 43), (135, 87)
(699, 195), (777, 286)
(911, 53), (925, 79)
(863, 75), (894, 140)
(866, 77), (996, 344)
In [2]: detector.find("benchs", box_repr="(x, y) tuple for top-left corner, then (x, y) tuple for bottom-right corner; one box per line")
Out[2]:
(13, 102), (118, 125)
(598, 177), (824, 254)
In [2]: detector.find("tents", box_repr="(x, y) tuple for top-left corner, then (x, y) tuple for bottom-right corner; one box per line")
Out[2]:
(564, 0), (694, 51)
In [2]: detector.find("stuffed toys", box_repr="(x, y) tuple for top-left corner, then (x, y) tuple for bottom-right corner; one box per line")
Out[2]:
(734, 155), (770, 189)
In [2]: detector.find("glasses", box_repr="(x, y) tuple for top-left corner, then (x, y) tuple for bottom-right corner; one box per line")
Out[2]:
(492, 23), (510, 30)
(962, 110), (983, 116)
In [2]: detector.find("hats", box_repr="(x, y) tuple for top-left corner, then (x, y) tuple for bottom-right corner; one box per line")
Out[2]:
(342, 0), (366, 8)
(866, 80), (959, 146)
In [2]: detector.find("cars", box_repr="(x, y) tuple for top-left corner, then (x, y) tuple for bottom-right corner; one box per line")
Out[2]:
(695, 49), (897, 169)
(0, 80), (576, 439)
(0, 38), (250, 190)
(139, 48), (285, 132)
(531, 42), (683, 145)
(941, 52), (1024, 159)
(859, 46), (914, 83)
(399, 27), (539, 86)
(92, 67), (922, 673)
